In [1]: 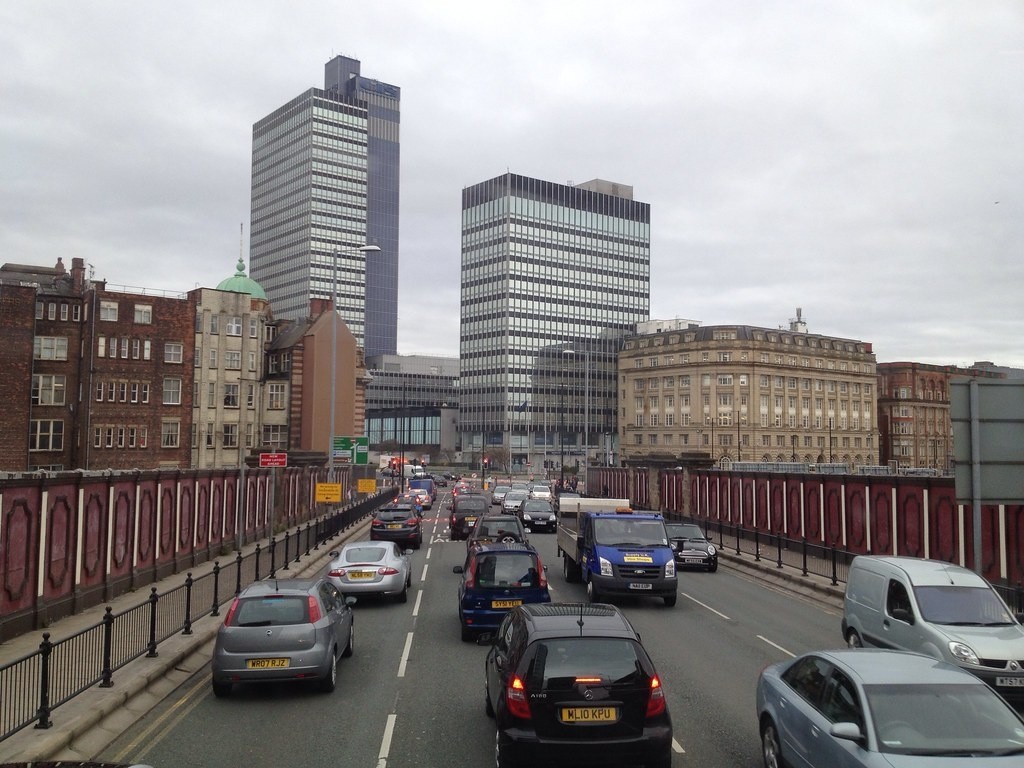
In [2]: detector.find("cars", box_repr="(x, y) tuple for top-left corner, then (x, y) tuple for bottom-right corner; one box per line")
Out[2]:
(377, 452), (587, 511)
(324, 540), (414, 604)
(407, 488), (434, 511)
(486, 601), (673, 768)
(393, 493), (425, 517)
(663, 522), (719, 574)
(210, 577), (359, 697)
(757, 645), (1024, 767)
(514, 498), (557, 534)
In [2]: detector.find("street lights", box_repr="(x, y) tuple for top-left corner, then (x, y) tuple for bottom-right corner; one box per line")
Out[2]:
(563, 350), (590, 496)
(327, 242), (382, 483)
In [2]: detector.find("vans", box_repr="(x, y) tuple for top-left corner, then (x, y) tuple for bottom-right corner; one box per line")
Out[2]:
(840, 555), (1023, 712)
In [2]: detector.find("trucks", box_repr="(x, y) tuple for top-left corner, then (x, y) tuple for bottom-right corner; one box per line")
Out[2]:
(555, 496), (685, 608)
(400, 464), (426, 480)
(378, 455), (410, 474)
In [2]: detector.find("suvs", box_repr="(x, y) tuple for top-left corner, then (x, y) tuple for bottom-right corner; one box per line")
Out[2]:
(370, 502), (425, 550)
(445, 495), (493, 540)
(454, 539), (553, 643)
(464, 514), (533, 556)
